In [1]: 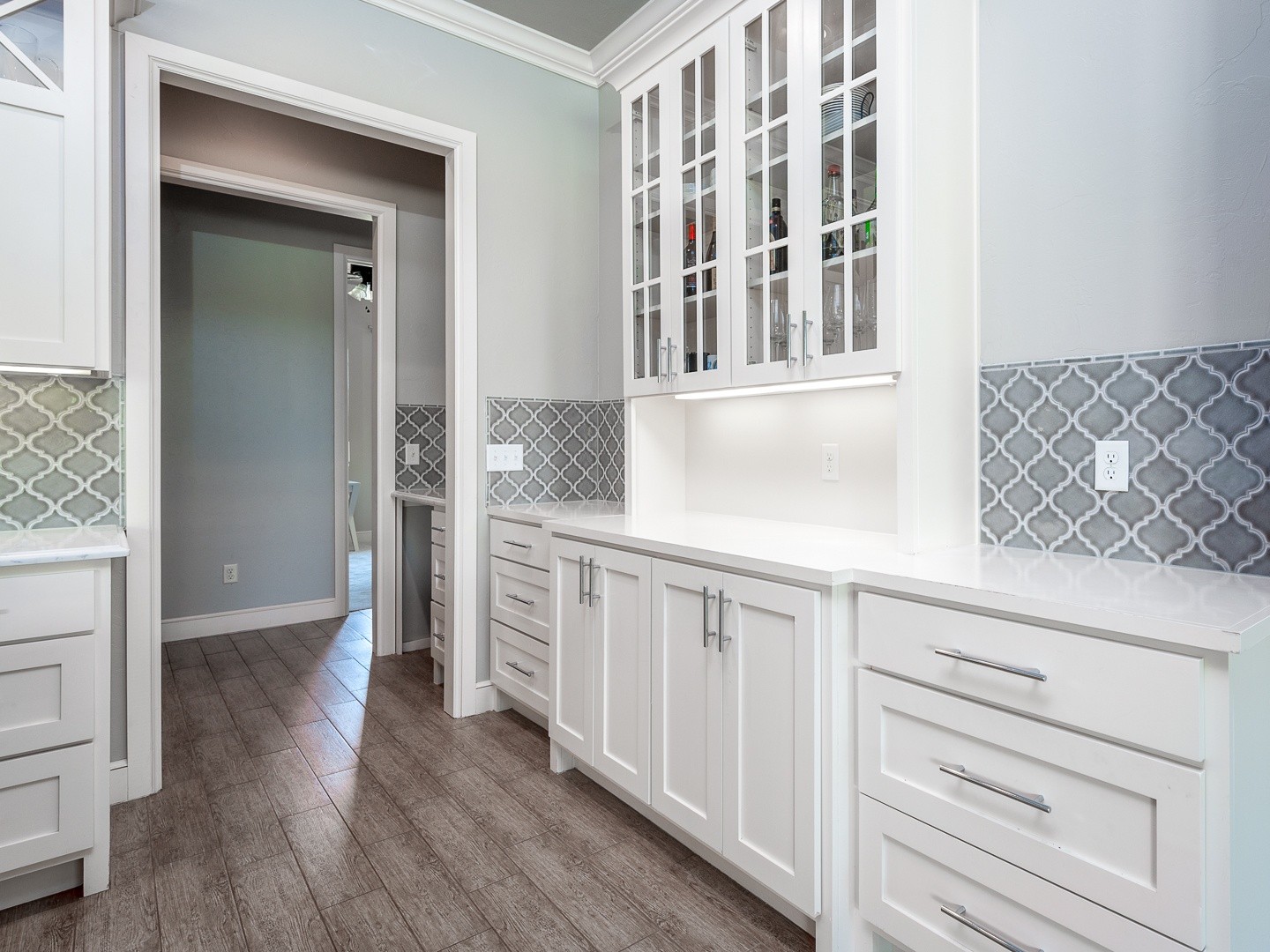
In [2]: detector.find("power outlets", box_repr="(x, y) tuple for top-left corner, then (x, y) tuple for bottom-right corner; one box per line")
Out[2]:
(223, 563), (239, 585)
(1095, 440), (1130, 493)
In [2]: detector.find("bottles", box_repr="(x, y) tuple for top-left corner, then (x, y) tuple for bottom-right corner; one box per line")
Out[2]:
(683, 224), (696, 297)
(865, 167), (877, 248)
(768, 198), (788, 275)
(822, 164), (844, 261)
(852, 189), (860, 253)
(705, 218), (716, 291)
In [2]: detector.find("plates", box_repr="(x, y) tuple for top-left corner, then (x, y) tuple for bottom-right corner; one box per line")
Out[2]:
(683, 168), (716, 196)
(821, 82), (874, 138)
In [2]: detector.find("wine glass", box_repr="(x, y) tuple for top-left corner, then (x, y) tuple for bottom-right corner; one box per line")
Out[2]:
(823, 279), (876, 355)
(768, 299), (788, 361)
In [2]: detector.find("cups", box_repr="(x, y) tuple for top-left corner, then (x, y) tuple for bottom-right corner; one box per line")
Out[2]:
(687, 352), (710, 372)
(707, 355), (717, 370)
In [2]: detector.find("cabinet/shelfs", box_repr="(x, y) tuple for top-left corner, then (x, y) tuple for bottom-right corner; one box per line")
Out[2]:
(0, 0), (113, 380)
(549, 536), (653, 806)
(856, 592), (1270, 951)
(489, 517), (549, 717)
(622, 0), (975, 401)
(650, 555), (826, 918)
(431, 505), (446, 684)
(0, 562), (109, 909)
